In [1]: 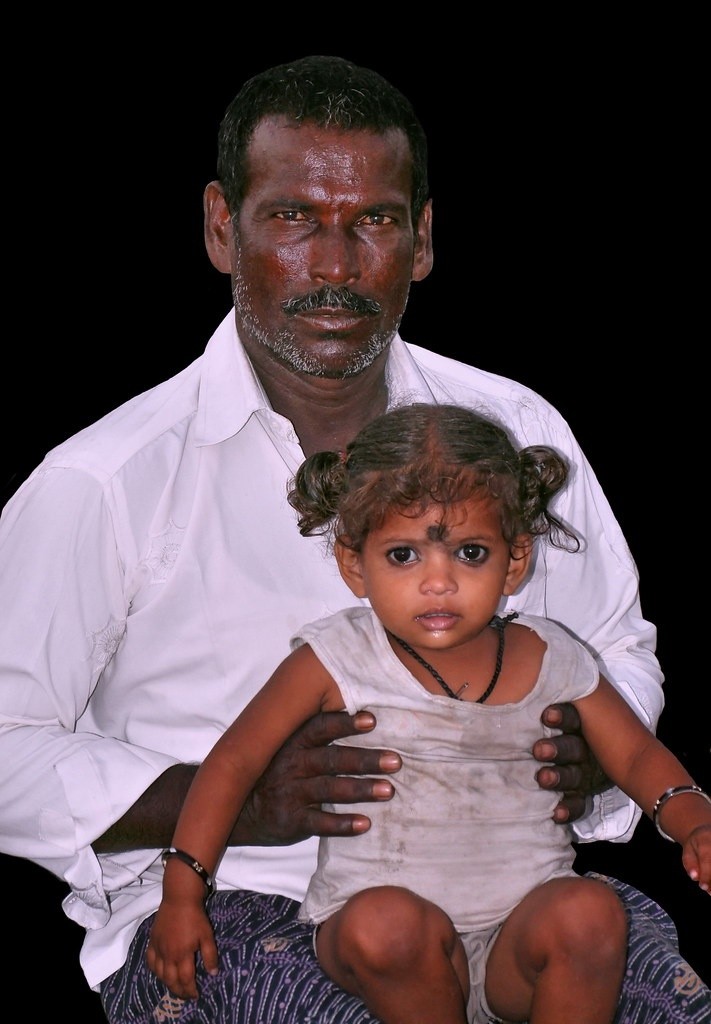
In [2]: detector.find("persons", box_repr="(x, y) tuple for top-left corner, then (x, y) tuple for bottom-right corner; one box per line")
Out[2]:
(146, 406), (711, 1024)
(0, 58), (710, 1023)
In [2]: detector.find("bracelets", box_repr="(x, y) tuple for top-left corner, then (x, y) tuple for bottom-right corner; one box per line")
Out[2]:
(162, 848), (214, 900)
(652, 785), (710, 844)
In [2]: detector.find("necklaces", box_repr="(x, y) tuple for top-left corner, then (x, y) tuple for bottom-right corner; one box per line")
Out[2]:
(390, 609), (519, 707)
